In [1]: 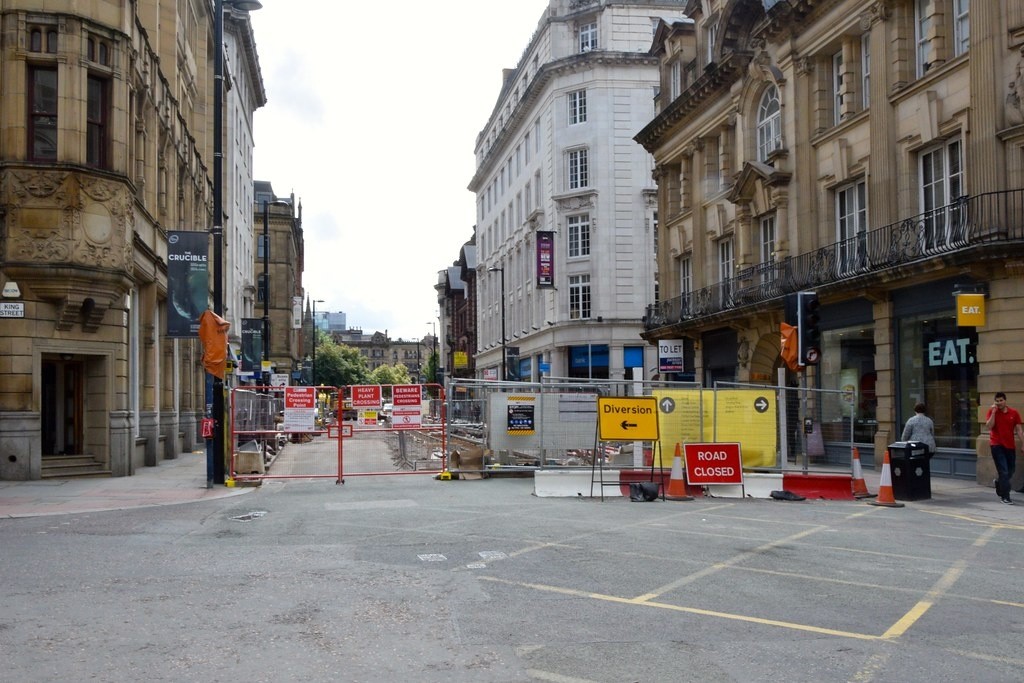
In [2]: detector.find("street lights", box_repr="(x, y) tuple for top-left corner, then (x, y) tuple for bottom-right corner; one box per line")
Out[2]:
(263, 200), (289, 396)
(313, 299), (325, 388)
(212, 0), (265, 484)
(426, 322), (436, 417)
(411, 337), (420, 384)
(488, 266), (506, 394)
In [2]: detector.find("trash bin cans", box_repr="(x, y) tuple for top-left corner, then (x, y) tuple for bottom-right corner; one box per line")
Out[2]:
(887, 441), (932, 500)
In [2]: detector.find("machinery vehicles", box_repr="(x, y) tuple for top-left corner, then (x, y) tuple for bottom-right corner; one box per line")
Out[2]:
(315, 393), (387, 428)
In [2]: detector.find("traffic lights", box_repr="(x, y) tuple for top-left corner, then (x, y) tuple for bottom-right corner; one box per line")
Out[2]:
(797, 290), (822, 366)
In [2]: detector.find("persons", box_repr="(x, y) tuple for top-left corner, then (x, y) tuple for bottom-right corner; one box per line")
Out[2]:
(474, 404), (481, 422)
(986, 392), (1024, 505)
(455, 399), (463, 418)
(901, 402), (935, 459)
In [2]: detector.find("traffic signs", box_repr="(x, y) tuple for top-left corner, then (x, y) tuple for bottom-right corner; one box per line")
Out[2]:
(596, 396), (661, 440)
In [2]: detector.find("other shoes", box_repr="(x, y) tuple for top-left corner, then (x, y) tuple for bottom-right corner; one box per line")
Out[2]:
(1001, 498), (1013, 504)
(993, 479), (1000, 495)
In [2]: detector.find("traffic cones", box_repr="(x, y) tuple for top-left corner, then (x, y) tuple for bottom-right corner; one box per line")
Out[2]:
(657, 442), (695, 501)
(849, 447), (878, 499)
(866, 451), (907, 508)
